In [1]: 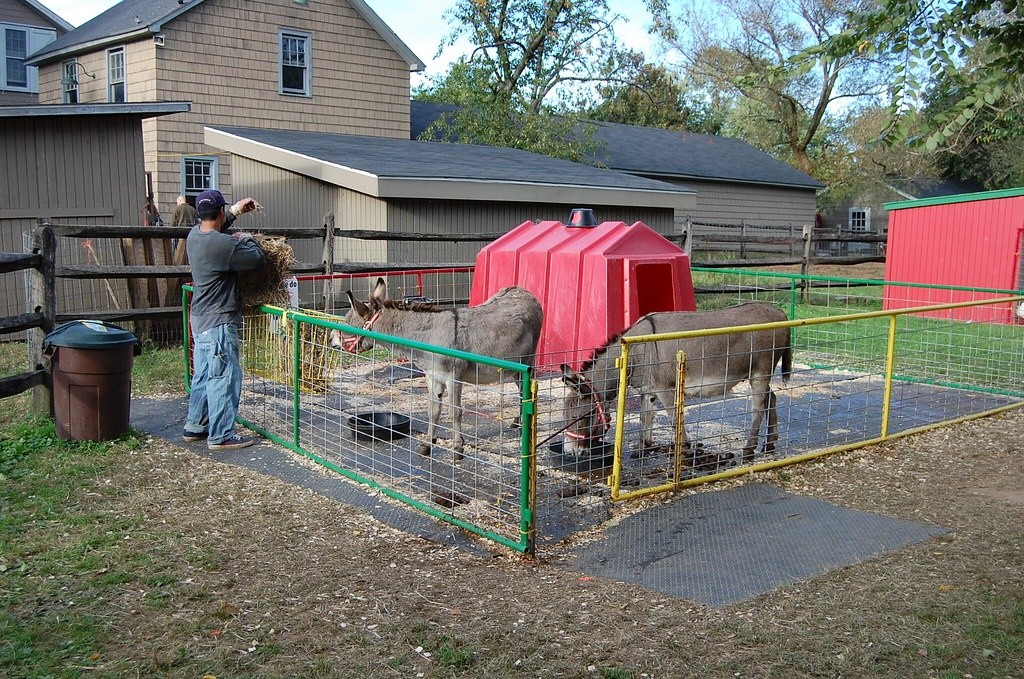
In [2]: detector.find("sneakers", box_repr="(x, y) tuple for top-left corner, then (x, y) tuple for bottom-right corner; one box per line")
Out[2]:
(208, 434), (254, 450)
(183, 428), (210, 442)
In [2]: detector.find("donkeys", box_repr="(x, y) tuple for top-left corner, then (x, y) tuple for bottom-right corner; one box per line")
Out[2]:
(560, 300), (795, 461)
(327, 277), (544, 461)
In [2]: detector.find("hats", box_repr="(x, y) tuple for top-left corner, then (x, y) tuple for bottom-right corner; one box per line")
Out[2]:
(195, 189), (229, 212)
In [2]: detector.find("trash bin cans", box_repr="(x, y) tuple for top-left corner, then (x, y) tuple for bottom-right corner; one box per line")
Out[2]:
(44, 319), (138, 444)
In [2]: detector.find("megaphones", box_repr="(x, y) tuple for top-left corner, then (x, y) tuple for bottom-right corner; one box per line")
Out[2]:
(153, 34), (165, 46)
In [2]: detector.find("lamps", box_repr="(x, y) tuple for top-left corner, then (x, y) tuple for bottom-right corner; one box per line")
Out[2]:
(60, 62), (96, 85)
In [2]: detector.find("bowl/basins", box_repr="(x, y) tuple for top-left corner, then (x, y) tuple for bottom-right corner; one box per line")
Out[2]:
(347, 413), (411, 441)
(546, 440), (619, 472)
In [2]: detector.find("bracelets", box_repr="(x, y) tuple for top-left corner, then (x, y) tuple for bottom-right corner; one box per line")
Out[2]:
(231, 206), (238, 215)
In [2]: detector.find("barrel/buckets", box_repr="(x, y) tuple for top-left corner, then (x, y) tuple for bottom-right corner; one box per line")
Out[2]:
(43, 320), (138, 442)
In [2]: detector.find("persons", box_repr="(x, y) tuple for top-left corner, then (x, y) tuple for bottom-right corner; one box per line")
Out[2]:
(172, 196), (197, 253)
(182, 189), (264, 450)
